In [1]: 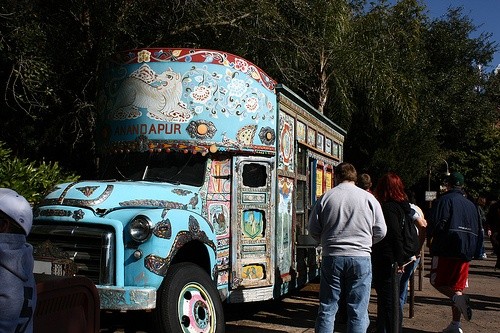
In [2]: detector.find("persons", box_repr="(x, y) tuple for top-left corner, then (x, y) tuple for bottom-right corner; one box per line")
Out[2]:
(355, 173), (373, 194)
(372, 171), (420, 333)
(398, 187), (428, 310)
(308, 161), (388, 333)
(425, 170), (484, 333)
(475, 192), (500, 269)
(0, 187), (37, 333)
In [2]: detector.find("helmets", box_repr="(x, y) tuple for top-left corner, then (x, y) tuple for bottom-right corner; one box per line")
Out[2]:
(0, 188), (33, 236)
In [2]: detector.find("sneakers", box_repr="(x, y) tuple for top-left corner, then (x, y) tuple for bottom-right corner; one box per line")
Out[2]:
(442, 320), (463, 333)
(454, 295), (472, 321)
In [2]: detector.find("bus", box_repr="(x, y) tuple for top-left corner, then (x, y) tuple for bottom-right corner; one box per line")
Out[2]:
(27, 47), (348, 333)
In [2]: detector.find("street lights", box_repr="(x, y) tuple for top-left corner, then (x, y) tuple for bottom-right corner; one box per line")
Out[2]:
(427, 157), (451, 208)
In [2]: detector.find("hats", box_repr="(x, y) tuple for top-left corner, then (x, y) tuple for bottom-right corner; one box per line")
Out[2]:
(445, 171), (465, 186)
